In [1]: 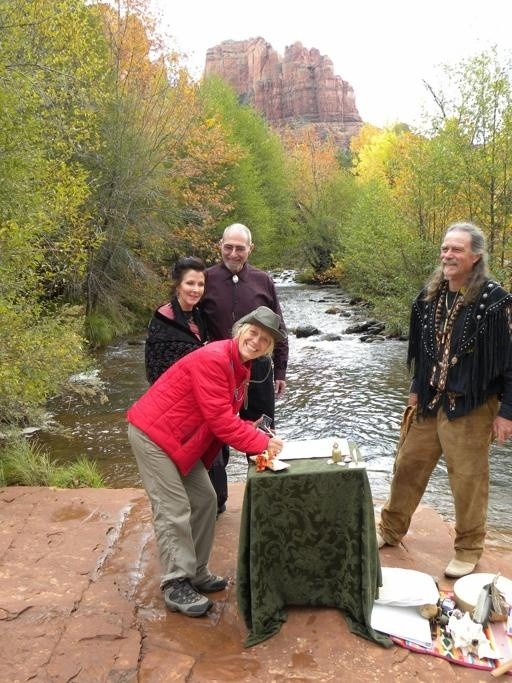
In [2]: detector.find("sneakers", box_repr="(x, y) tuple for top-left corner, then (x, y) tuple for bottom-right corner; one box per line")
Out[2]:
(216, 504), (226, 521)
(187, 572), (227, 592)
(164, 582), (212, 618)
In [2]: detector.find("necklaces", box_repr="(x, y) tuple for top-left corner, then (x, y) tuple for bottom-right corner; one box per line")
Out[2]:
(441, 283), (463, 344)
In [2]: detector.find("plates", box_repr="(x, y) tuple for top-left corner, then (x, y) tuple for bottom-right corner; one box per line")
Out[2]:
(249, 453), (291, 471)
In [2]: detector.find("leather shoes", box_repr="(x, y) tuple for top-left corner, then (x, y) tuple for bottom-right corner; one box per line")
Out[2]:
(375, 534), (394, 548)
(445, 559), (477, 578)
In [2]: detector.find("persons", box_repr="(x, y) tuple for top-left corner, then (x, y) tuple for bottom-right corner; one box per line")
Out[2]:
(375, 221), (512, 580)
(124, 303), (285, 615)
(140, 256), (228, 517)
(200, 220), (286, 456)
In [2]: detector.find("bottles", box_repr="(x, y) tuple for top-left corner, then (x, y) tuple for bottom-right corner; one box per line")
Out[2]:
(332, 442), (341, 464)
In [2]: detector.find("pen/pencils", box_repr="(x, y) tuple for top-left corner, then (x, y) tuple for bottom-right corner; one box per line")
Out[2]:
(264, 424), (276, 438)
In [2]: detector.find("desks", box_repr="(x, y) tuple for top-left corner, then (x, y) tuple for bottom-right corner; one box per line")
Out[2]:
(246, 438), (371, 607)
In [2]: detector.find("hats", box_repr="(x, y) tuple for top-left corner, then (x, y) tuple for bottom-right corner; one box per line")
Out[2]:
(241, 306), (288, 342)
